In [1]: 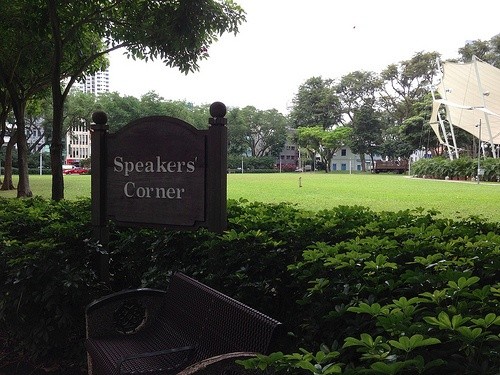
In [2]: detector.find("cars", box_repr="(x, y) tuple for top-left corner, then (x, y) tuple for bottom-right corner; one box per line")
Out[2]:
(295, 164), (319, 174)
(64, 166), (88, 175)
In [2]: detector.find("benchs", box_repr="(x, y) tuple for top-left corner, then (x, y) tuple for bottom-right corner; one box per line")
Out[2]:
(84, 271), (283, 375)
(373, 160), (409, 174)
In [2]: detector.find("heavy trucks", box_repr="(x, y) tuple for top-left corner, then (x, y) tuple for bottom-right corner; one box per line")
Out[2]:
(367, 159), (409, 174)
